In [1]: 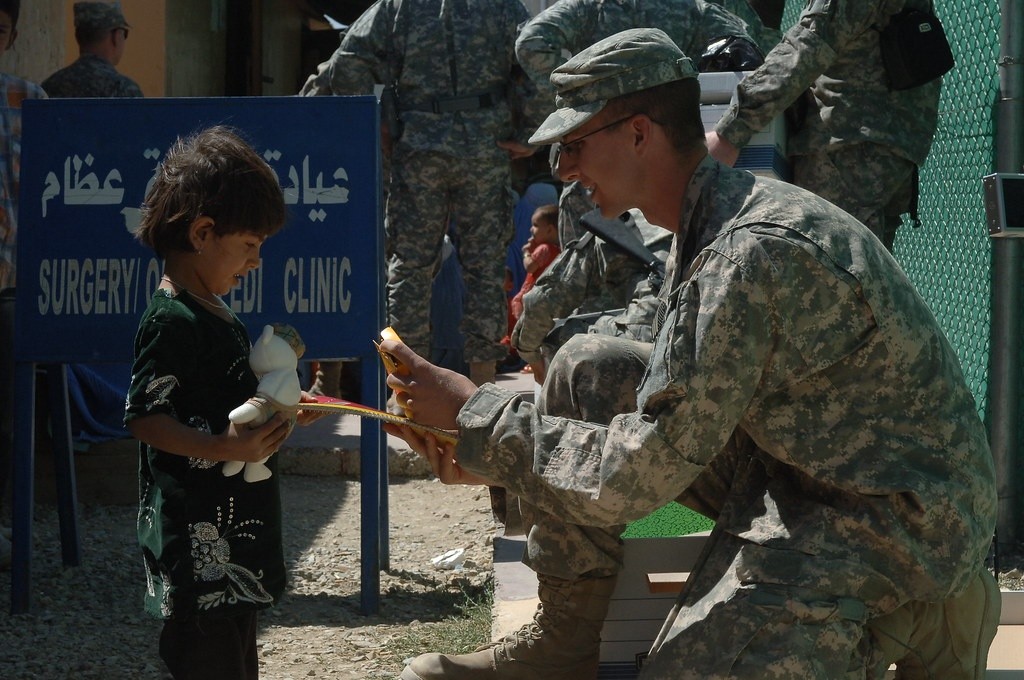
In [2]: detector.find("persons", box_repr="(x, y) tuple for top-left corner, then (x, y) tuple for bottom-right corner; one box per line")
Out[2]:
(122, 127), (327, 680)
(40, 1), (145, 98)
(0, 0), (51, 568)
(702, 0), (958, 251)
(378, 28), (1001, 680)
(300, 0), (761, 416)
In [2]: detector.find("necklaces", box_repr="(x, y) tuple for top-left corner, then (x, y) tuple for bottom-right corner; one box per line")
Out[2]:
(160, 277), (225, 309)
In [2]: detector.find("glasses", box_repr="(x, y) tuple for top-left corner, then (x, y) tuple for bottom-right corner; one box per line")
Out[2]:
(110, 27), (128, 40)
(559, 112), (665, 158)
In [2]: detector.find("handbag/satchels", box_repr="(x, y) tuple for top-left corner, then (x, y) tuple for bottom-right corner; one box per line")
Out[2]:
(879, 10), (955, 91)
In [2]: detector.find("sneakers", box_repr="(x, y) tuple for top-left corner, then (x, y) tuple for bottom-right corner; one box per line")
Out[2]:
(386, 391), (405, 416)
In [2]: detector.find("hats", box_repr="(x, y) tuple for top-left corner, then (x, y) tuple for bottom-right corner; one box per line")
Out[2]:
(74, 1), (134, 30)
(528, 28), (699, 147)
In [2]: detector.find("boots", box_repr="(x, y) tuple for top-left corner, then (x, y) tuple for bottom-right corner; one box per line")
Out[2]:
(306, 363), (342, 400)
(869, 566), (1002, 680)
(402, 572), (617, 680)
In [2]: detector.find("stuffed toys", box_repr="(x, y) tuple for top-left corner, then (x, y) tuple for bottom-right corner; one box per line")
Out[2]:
(221, 323), (305, 482)
